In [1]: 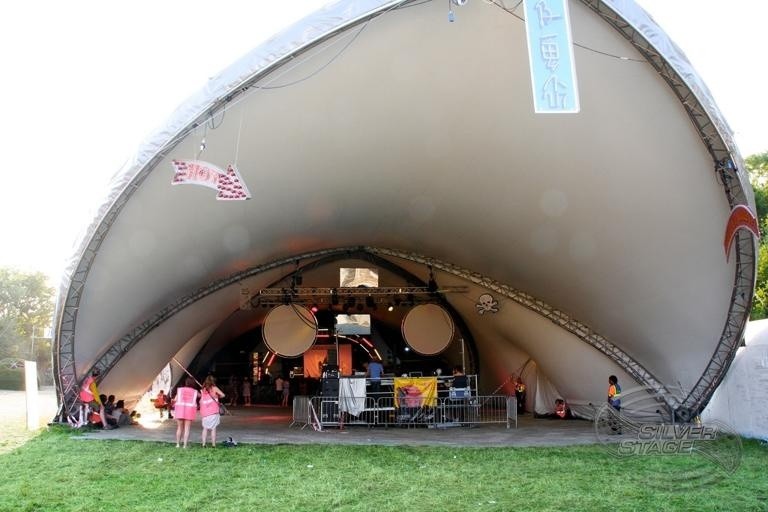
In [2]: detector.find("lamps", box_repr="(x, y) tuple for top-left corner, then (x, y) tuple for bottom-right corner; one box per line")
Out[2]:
(258, 267), (439, 313)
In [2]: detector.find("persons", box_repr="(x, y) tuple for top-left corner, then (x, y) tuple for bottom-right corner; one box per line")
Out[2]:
(364, 355), (385, 403)
(396, 385), (424, 408)
(513, 378), (527, 415)
(451, 364), (470, 422)
(553, 398), (568, 419)
(80, 368), (291, 450)
(607, 375), (622, 435)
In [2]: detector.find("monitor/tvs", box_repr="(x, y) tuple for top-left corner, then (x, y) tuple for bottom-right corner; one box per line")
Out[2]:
(334, 313), (371, 335)
